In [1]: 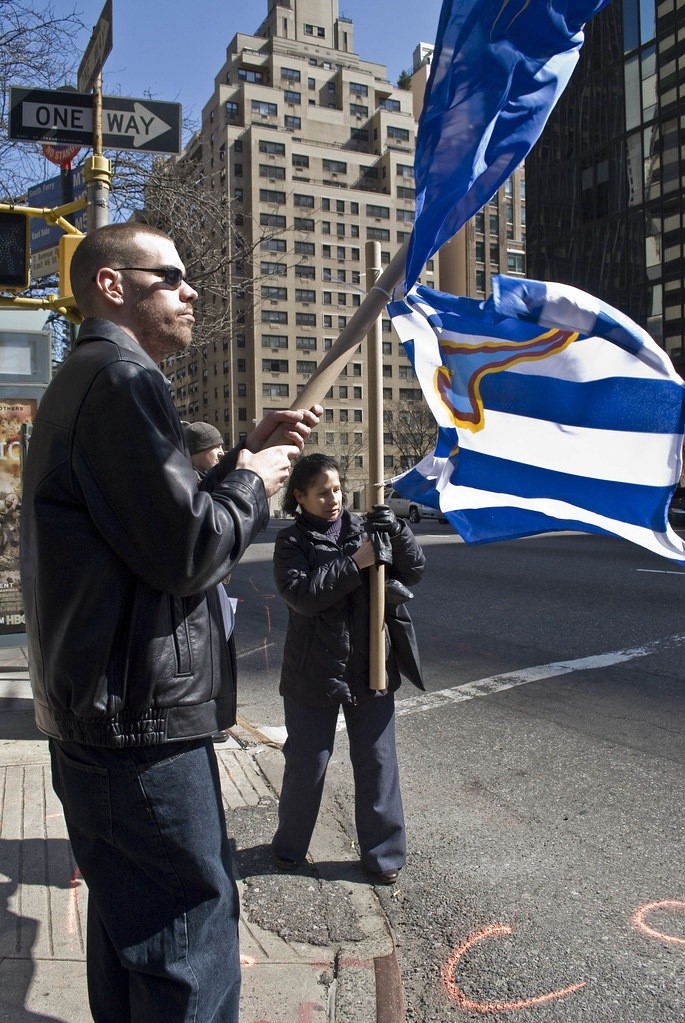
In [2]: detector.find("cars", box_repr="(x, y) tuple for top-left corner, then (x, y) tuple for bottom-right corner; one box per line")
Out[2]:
(385, 487), (450, 525)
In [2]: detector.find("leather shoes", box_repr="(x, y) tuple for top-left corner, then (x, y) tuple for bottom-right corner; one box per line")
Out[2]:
(271, 833), (299, 871)
(375, 868), (399, 883)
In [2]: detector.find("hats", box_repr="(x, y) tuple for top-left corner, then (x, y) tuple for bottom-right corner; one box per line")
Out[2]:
(185, 421), (224, 456)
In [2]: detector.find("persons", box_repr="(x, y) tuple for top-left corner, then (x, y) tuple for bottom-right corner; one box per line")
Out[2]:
(18, 222), (323, 1023)
(270, 452), (427, 882)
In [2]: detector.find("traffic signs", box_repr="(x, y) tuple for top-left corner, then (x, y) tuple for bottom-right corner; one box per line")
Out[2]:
(7, 85), (183, 154)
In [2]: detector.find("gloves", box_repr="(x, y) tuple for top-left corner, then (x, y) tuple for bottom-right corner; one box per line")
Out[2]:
(364, 504), (401, 537)
(369, 531), (393, 565)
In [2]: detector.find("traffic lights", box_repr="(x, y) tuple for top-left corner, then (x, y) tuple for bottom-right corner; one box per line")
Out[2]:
(0, 212), (31, 292)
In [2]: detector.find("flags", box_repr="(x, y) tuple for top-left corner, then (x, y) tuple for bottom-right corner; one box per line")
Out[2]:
(383, 274), (685, 566)
(403, 0), (612, 298)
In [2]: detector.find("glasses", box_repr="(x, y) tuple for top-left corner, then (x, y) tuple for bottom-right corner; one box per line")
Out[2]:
(90, 266), (189, 290)
